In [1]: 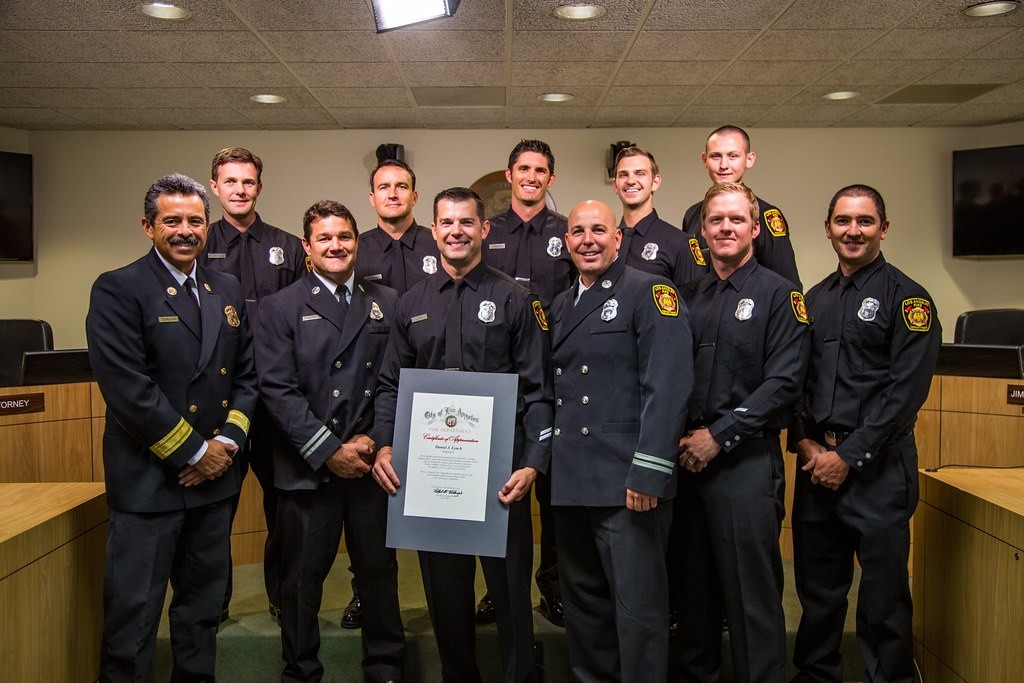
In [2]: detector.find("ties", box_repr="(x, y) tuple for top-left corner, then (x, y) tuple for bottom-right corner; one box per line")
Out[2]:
(239, 233), (257, 309)
(813, 276), (850, 422)
(445, 280), (466, 371)
(183, 277), (198, 307)
(515, 222), (532, 288)
(686, 280), (727, 430)
(336, 285), (348, 313)
(392, 240), (406, 296)
(618, 227), (634, 264)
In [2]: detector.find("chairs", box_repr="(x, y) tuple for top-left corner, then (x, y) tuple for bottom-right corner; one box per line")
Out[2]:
(956, 309), (1023, 348)
(0, 318), (56, 387)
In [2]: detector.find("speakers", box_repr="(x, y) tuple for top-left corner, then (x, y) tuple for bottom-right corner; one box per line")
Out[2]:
(611, 141), (636, 171)
(376, 143), (404, 165)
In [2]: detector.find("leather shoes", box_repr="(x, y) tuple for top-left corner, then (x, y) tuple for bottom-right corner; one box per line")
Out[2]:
(540, 593), (565, 626)
(477, 595), (498, 625)
(269, 604), (281, 620)
(341, 596), (363, 628)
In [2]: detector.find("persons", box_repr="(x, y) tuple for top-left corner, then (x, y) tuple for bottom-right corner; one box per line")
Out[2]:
(672, 182), (812, 683)
(475, 140), (580, 627)
(547, 200), (696, 683)
(786, 184), (944, 683)
(196, 146), (308, 622)
(85, 172), (261, 683)
(683, 124), (802, 629)
(613, 147), (708, 627)
(253, 200), (409, 683)
(342, 160), (442, 629)
(372, 188), (554, 683)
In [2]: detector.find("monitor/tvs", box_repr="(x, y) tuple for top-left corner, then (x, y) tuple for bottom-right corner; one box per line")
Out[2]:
(0, 150), (34, 264)
(20, 349), (97, 386)
(934, 344), (1024, 380)
(952, 144), (1024, 257)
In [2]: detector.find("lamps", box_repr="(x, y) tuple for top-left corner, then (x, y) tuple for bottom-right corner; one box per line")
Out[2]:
(372, 0), (461, 33)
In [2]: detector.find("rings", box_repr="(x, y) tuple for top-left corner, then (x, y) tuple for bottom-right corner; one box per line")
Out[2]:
(688, 459), (694, 464)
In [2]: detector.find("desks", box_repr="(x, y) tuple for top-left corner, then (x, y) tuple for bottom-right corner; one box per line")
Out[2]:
(911, 464), (1024, 683)
(0, 482), (112, 683)
